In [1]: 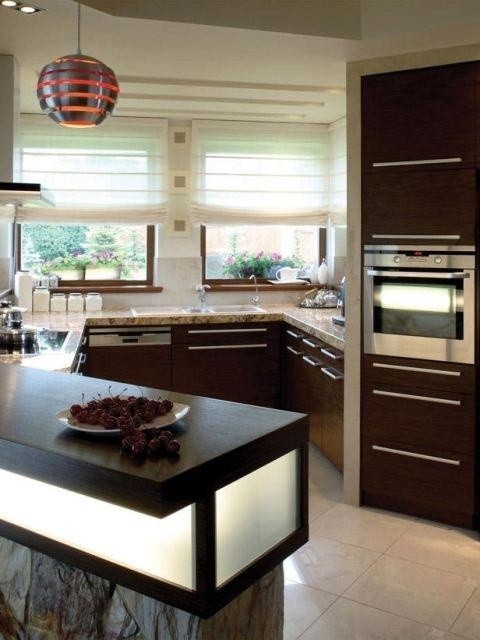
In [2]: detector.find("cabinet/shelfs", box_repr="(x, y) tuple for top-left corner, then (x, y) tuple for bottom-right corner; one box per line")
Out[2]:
(87, 325), (172, 392)
(171, 324), (281, 411)
(280, 321), (345, 475)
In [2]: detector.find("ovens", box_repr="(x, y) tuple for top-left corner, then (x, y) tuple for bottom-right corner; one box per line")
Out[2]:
(363, 242), (476, 363)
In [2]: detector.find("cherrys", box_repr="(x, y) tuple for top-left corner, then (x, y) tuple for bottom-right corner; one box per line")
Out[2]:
(70, 384), (181, 467)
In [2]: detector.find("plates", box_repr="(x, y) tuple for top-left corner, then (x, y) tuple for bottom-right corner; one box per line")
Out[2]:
(56, 396), (190, 433)
(266, 279), (308, 284)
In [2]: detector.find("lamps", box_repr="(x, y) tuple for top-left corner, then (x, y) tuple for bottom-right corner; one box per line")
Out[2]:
(36, 3), (120, 129)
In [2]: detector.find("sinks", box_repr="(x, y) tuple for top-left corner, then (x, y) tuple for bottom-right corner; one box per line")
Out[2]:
(180, 305), (210, 313)
(0, 326), (69, 356)
(210, 305), (266, 312)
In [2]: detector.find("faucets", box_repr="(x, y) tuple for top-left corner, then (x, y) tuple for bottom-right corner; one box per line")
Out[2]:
(195, 283), (208, 307)
(249, 274), (261, 306)
(0, 288), (29, 315)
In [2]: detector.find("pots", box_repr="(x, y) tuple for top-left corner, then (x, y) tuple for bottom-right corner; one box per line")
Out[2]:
(0, 299), (38, 346)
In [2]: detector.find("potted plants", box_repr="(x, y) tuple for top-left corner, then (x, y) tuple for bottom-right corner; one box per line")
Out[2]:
(42, 254), (85, 280)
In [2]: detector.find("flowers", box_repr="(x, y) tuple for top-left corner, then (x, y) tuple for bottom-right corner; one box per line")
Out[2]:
(82, 250), (131, 278)
(223, 251), (304, 280)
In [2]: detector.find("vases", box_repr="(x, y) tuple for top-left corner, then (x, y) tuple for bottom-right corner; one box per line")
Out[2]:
(85, 265), (123, 280)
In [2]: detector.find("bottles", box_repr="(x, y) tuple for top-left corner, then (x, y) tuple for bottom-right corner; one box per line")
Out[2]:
(50, 292), (66, 312)
(14, 270), (32, 312)
(32, 285), (50, 312)
(67, 292), (84, 312)
(85, 292), (103, 311)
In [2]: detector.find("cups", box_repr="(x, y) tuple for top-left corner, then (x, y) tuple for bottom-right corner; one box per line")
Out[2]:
(276, 268), (298, 282)
